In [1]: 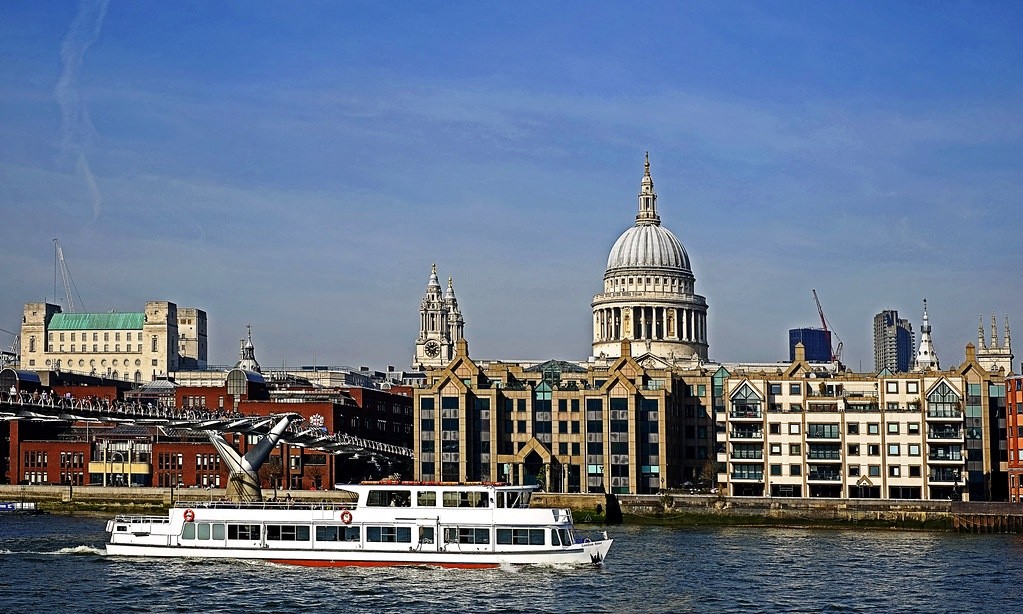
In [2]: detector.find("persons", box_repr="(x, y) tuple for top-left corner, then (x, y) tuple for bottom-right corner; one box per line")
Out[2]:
(217, 493), (357, 510)
(72, 481), (77, 486)
(310, 485), (316, 491)
(0, 384), (98, 411)
(95, 398), (187, 419)
(183, 405), (277, 428)
(539, 486), (553, 493)
(332, 534), (355, 542)
(284, 422), (359, 446)
(389, 496), (422, 507)
(277, 485), (283, 491)
(202, 483), (228, 490)
(585, 512), (592, 523)
(324, 487), (328, 492)
(458, 497), (488, 507)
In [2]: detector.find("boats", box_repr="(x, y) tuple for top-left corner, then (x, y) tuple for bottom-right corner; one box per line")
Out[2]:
(105, 414), (615, 571)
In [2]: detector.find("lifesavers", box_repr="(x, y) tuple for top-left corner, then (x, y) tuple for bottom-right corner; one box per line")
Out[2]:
(341, 511), (352, 524)
(584, 538), (591, 543)
(184, 509), (194, 522)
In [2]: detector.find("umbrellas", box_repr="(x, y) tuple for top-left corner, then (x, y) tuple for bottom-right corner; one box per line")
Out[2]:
(684, 481), (707, 485)
